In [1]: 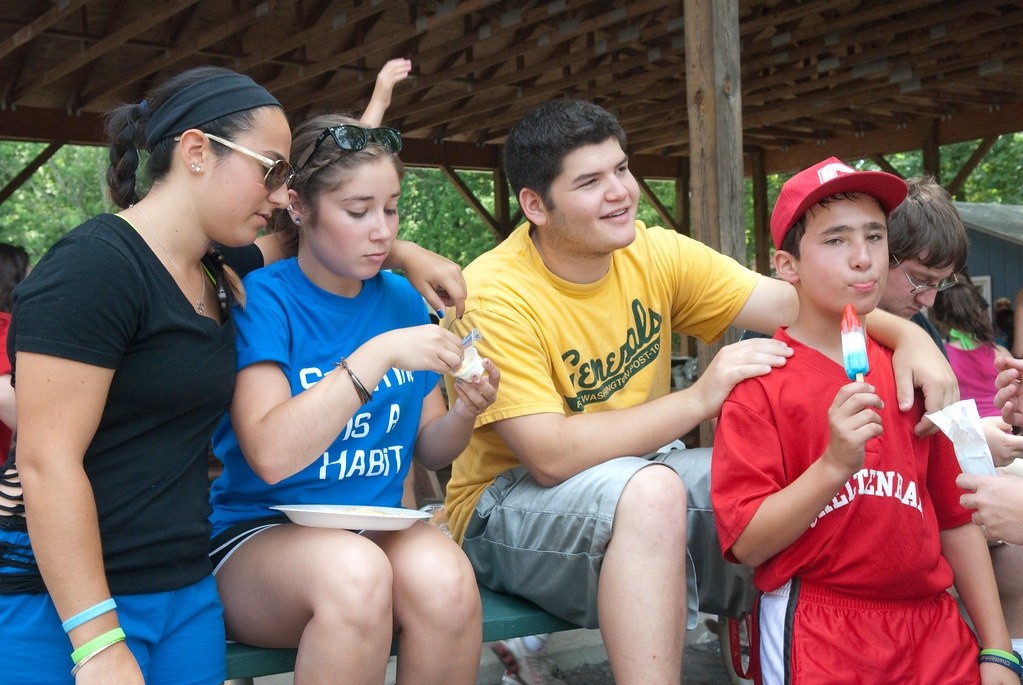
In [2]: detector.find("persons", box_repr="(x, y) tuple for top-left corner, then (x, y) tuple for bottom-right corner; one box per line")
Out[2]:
(0, 59), (468, 685)
(439, 101), (962, 685)
(709, 157), (1023, 685)
(207, 112), (502, 685)
(737, 174), (1023, 653)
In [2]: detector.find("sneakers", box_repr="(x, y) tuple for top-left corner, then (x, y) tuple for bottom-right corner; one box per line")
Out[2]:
(490, 637), (553, 685)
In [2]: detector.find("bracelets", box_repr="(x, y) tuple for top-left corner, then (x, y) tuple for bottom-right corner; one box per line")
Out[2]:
(979, 649), (1023, 678)
(70, 638), (125, 677)
(62, 598), (118, 633)
(71, 627), (127, 663)
(332, 357), (372, 404)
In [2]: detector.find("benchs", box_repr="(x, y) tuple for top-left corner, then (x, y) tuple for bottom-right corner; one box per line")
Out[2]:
(222, 581), (584, 679)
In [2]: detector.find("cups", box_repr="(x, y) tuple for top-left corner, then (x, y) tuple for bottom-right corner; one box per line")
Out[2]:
(451, 347), (485, 385)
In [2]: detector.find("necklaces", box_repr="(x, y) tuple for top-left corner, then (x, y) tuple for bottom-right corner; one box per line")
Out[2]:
(129, 205), (205, 314)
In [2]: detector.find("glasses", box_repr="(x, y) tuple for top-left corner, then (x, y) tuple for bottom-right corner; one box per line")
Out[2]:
(172, 129), (302, 192)
(892, 254), (958, 295)
(292, 125), (403, 174)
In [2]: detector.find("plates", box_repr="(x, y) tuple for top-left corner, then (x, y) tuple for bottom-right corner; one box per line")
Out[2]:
(268, 504), (434, 531)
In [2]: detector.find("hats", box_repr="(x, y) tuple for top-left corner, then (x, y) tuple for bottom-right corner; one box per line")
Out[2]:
(769, 156), (908, 251)
